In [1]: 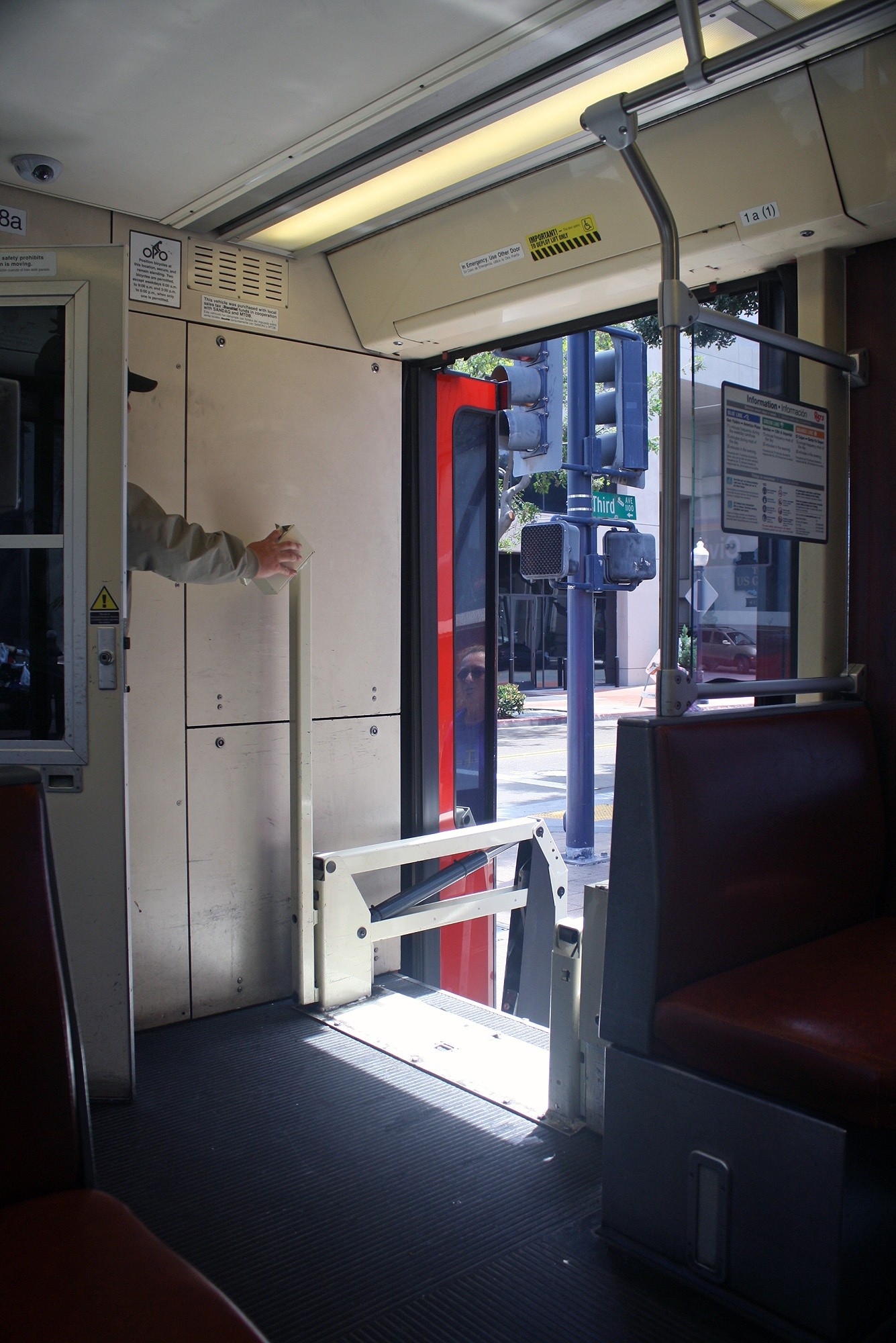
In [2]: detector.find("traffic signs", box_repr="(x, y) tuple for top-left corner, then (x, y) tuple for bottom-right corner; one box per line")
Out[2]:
(592, 492), (637, 519)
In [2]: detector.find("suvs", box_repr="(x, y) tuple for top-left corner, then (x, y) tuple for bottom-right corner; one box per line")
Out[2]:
(684, 624), (758, 674)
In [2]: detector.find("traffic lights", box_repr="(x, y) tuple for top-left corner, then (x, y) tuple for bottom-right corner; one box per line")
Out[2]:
(589, 326), (647, 473)
(489, 334), (563, 477)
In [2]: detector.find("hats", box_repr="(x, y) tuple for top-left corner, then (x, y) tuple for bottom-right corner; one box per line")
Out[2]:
(128, 365), (158, 393)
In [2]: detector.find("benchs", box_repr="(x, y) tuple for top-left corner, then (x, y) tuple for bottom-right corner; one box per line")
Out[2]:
(595, 712), (896, 1122)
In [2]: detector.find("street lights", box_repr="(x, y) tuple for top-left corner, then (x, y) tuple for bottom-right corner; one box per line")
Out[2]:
(690, 538), (710, 682)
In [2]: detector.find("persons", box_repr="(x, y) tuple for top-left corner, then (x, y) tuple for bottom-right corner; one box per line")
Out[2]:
(127, 368), (303, 585)
(453, 645), (484, 823)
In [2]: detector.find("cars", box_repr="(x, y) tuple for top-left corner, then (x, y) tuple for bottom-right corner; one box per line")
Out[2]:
(498, 641), (550, 672)
